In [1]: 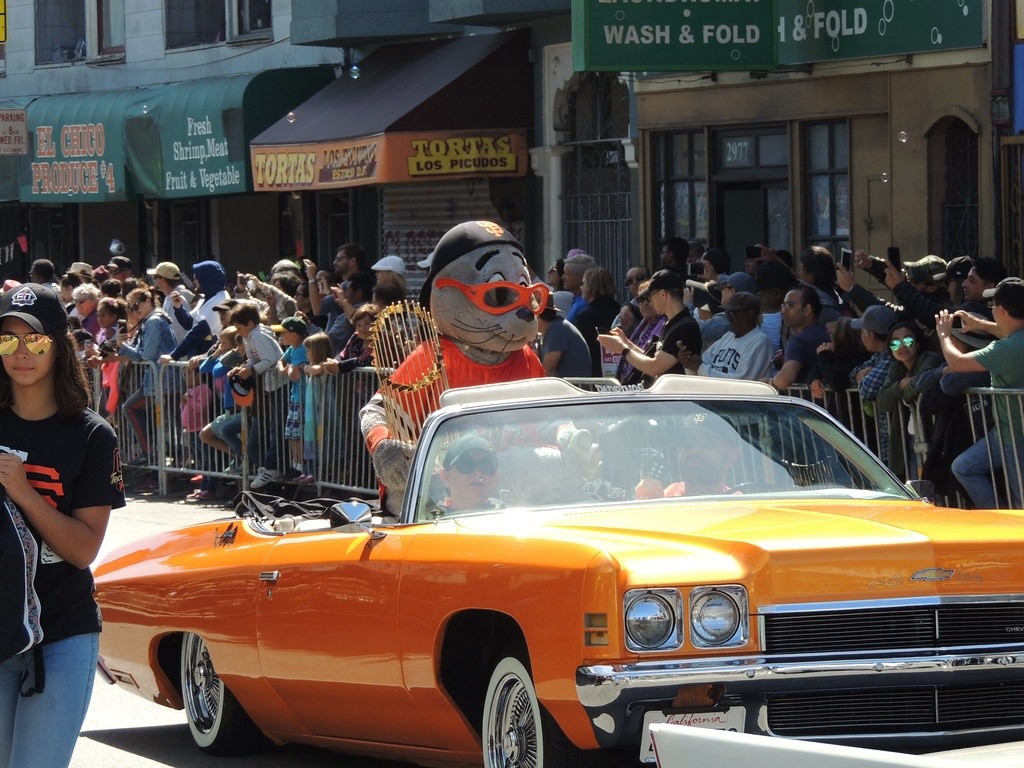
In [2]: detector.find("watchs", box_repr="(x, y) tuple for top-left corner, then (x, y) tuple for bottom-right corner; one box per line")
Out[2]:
(309, 279), (318, 284)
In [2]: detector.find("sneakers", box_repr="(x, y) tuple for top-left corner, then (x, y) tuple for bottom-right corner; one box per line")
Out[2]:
(119, 454), (303, 507)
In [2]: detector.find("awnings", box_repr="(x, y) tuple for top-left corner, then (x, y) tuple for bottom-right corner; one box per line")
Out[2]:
(249, 24), (529, 192)
(18, 64), (338, 203)
(0, 96), (40, 203)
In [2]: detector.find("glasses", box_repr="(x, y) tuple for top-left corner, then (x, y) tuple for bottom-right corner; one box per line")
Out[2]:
(153, 275), (160, 280)
(109, 269), (123, 275)
(625, 274), (646, 287)
(636, 295), (649, 305)
(335, 255), (347, 262)
(449, 457), (495, 475)
(987, 301), (994, 309)
(548, 267), (558, 274)
(779, 302), (794, 311)
(888, 336), (914, 352)
(0, 332), (55, 358)
(128, 302), (139, 313)
(73, 299), (86, 304)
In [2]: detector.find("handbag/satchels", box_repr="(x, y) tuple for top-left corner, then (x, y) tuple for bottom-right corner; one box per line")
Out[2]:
(0, 482), (46, 697)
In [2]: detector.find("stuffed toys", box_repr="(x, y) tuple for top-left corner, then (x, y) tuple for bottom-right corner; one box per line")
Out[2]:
(359, 220), (550, 523)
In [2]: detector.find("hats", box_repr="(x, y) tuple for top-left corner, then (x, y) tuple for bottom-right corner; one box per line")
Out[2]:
(850, 305), (897, 335)
(442, 434), (496, 468)
(535, 291), (564, 313)
(67, 262), (95, 280)
(212, 300), (238, 311)
(106, 257), (131, 269)
(416, 252), (434, 270)
(717, 292), (761, 311)
(0, 280), (24, 292)
(91, 265), (110, 281)
(932, 255), (975, 282)
(719, 272), (755, 302)
(147, 261), (181, 280)
(638, 270), (684, 298)
(682, 412), (734, 443)
(982, 277), (1024, 305)
(270, 317), (308, 335)
(371, 255), (406, 276)
(0, 283), (68, 333)
(903, 255), (948, 284)
(228, 374), (255, 407)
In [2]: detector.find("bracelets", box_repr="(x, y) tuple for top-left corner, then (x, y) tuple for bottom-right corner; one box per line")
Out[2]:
(621, 345), (631, 358)
(849, 284), (854, 292)
(769, 378), (777, 390)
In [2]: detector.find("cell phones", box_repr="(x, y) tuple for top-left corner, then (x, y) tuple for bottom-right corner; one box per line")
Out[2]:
(887, 247), (901, 273)
(239, 277), (249, 286)
(841, 248), (852, 273)
(118, 319), (127, 334)
(688, 263), (704, 276)
(745, 247), (762, 259)
(951, 315), (964, 330)
(595, 326), (609, 336)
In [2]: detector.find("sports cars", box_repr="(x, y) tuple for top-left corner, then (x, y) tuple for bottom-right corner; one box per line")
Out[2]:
(87, 373), (1024, 768)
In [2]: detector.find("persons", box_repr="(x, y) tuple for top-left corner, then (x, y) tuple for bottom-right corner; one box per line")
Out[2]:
(526, 237), (1024, 510)
(0, 241), (411, 504)
(433, 413), (743, 521)
(1, 281), (128, 768)
